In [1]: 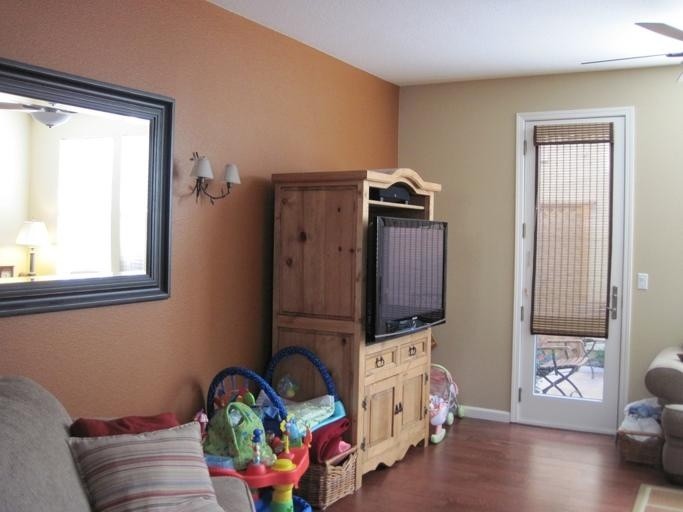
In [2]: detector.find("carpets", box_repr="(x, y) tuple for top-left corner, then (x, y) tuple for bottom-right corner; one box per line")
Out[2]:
(632, 483), (683, 512)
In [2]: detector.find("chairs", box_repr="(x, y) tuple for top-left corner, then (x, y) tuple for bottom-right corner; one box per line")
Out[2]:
(644, 346), (682, 483)
(0, 375), (255, 512)
(536, 339), (597, 398)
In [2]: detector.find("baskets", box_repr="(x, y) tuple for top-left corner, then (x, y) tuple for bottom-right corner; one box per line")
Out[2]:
(294, 450), (358, 512)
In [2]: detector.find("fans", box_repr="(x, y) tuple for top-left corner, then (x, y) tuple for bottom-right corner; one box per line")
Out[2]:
(581, 23), (683, 83)
(0, 91), (78, 114)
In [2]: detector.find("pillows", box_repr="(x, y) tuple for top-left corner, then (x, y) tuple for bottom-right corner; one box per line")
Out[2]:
(67, 413), (224, 512)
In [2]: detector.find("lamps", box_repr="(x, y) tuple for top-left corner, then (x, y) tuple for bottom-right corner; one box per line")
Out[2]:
(188, 152), (241, 204)
(30, 112), (71, 129)
(17, 221), (48, 281)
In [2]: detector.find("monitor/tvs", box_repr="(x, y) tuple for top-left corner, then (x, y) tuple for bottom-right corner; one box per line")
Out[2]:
(366, 215), (448, 344)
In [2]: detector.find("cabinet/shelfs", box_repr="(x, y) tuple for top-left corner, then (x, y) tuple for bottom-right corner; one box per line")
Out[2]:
(271, 168), (442, 491)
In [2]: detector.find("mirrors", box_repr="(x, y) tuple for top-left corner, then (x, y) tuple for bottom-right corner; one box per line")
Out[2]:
(0, 57), (177, 318)
